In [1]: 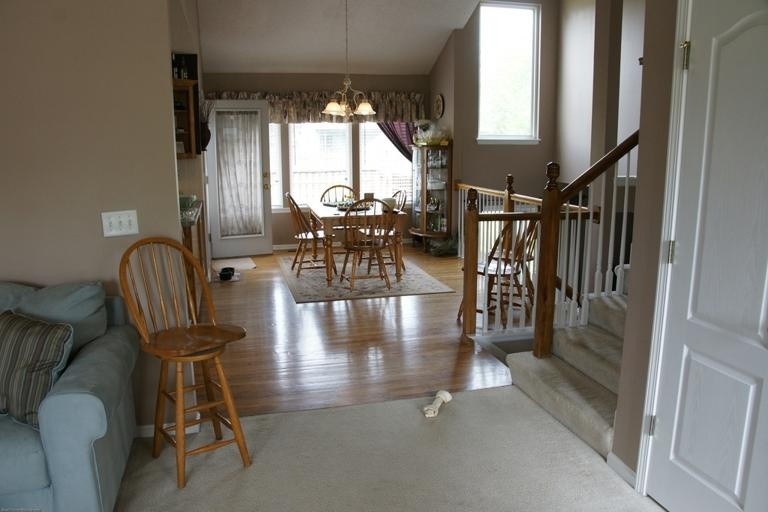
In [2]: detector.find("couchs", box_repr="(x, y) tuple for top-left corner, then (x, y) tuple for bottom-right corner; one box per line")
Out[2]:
(0, 282), (139, 510)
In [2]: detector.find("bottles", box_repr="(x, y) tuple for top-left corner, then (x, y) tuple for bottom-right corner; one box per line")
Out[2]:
(172, 52), (188, 79)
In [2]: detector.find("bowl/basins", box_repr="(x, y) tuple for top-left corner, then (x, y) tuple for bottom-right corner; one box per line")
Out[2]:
(179, 195), (196, 210)
(220, 267), (234, 280)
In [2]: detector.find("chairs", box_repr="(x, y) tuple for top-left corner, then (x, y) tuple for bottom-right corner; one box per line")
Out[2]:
(285, 184), (409, 293)
(120, 236), (252, 489)
(457, 218), (538, 330)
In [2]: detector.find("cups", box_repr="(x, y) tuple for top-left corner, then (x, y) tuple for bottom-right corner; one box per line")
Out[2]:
(382, 198), (396, 211)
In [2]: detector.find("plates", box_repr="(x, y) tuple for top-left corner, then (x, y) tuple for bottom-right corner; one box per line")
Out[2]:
(382, 210), (400, 214)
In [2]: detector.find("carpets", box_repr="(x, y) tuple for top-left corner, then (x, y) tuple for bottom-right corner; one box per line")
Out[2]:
(274, 250), (457, 304)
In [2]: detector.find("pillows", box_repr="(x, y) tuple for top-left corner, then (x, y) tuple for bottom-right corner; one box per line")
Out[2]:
(1, 307), (74, 432)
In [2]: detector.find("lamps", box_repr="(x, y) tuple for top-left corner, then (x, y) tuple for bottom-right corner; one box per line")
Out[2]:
(319, 0), (378, 118)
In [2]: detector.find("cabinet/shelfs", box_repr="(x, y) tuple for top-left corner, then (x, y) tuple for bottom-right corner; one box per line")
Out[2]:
(173, 78), (198, 161)
(408, 144), (453, 254)
